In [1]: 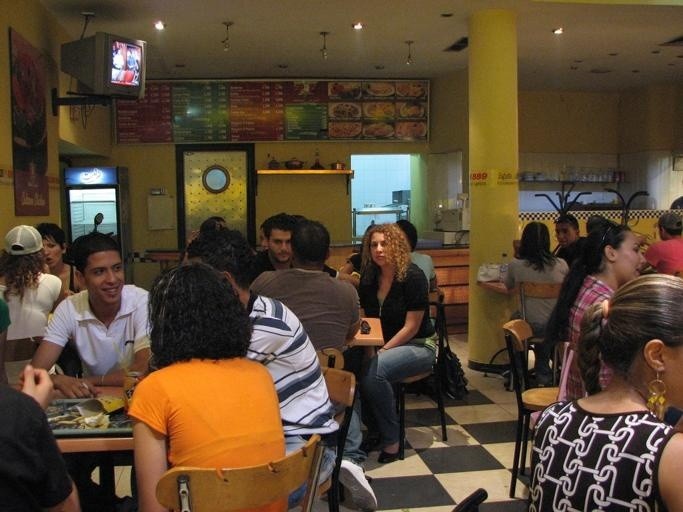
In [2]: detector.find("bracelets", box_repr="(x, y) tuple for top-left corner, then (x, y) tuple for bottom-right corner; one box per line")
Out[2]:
(98, 375), (105, 385)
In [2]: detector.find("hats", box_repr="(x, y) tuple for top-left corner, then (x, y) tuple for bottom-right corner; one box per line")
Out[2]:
(658, 213), (683, 230)
(4, 225), (43, 256)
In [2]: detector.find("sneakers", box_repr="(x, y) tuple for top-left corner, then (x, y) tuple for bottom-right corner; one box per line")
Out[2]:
(339, 460), (377, 511)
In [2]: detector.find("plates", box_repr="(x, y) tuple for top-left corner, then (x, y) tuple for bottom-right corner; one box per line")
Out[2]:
(518, 169), (546, 182)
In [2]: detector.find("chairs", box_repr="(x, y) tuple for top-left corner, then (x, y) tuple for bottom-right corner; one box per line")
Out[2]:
(152, 345), (356, 512)
(503, 276), (569, 498)
(388, 291), (448, 460)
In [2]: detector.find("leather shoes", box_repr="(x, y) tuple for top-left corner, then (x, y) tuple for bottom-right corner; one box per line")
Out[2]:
(378, 449), (399, 463)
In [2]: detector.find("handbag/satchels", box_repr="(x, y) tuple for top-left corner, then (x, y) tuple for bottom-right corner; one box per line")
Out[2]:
(477, 264), (508, 282)
(444, 345), (468, 400)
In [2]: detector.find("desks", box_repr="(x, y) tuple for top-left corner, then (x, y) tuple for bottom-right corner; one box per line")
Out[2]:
(476, 279), (518, 388)
(339, 317), (385, 357)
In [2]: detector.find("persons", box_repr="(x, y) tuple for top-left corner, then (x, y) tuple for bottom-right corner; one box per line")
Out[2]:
(128, 261), (285, 511)
(359, 224), (438, 463)
(182, 216), (340, 511)
(0, 297), (81, 511)
(126, 50), (136, 69)
(0, 223), (81, 340)
(133, 59), (140, 83)
(33, 232), (154, 512)
(249, 220), (378, 510)
(113, 47), (124, 68)
(187, 210), (438, 292)
(526, 271), (683, 512)
(505, 209), (683, 403)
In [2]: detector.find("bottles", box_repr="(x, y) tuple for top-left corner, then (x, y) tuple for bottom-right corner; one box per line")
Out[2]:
(498, 253), (509, 289)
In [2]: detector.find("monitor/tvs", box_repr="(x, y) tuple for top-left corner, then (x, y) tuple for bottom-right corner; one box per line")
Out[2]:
(59, 30), (148, 100)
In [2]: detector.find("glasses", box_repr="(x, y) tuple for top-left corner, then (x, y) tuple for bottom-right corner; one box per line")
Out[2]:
(603, 219), (618, 241)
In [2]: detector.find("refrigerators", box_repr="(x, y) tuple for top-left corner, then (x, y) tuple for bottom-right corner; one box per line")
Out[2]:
(65, 165), (130, 290)
